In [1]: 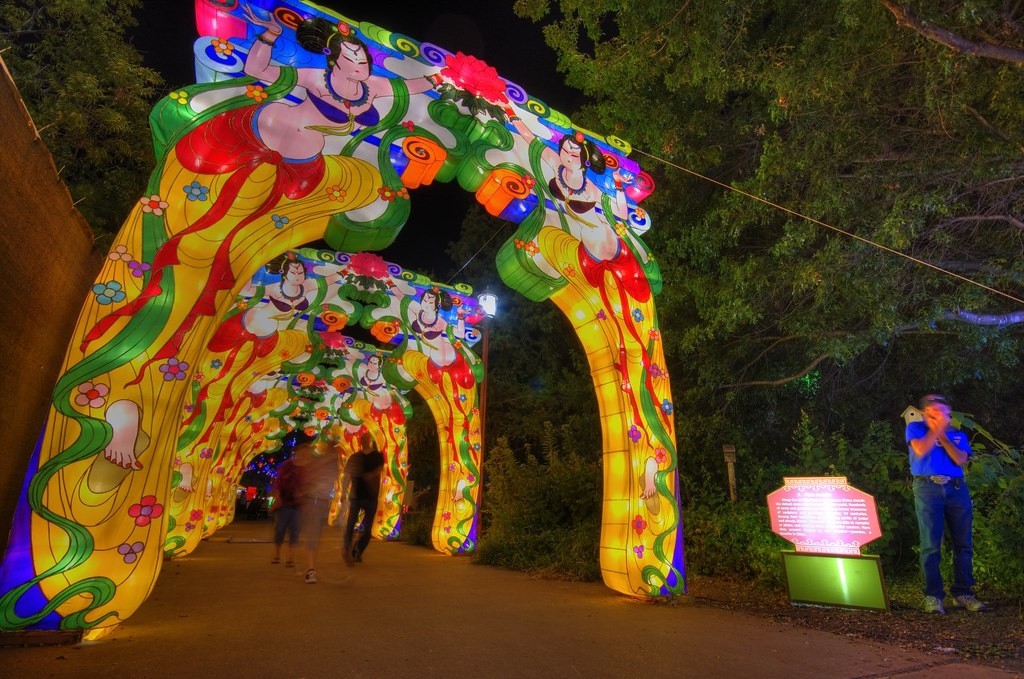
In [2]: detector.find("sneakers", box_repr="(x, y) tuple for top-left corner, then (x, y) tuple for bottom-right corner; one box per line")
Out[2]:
(918, 595), (945, 614)
(952, 594), (986, 612)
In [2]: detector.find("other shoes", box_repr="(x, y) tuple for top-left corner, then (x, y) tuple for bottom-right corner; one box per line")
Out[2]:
(271, 557), (280, 562)
(286, 560), (294, 567)
(303, 569), (317, 583)
(347, 548), (362, 563)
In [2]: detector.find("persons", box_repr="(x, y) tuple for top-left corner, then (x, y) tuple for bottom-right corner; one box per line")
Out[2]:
(266, 429), (384, 585)
(906, 394), (986, 616)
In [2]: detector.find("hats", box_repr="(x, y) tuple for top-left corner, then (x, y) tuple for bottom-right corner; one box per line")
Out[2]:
(319, 434), (336, 443)
(919, 394), (949, 408)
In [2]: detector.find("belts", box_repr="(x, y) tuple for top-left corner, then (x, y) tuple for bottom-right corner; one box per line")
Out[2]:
(913, 475), (962, 483)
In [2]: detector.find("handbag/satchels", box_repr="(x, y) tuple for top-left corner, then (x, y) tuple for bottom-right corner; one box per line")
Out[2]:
(267, 484), (292, 512)
